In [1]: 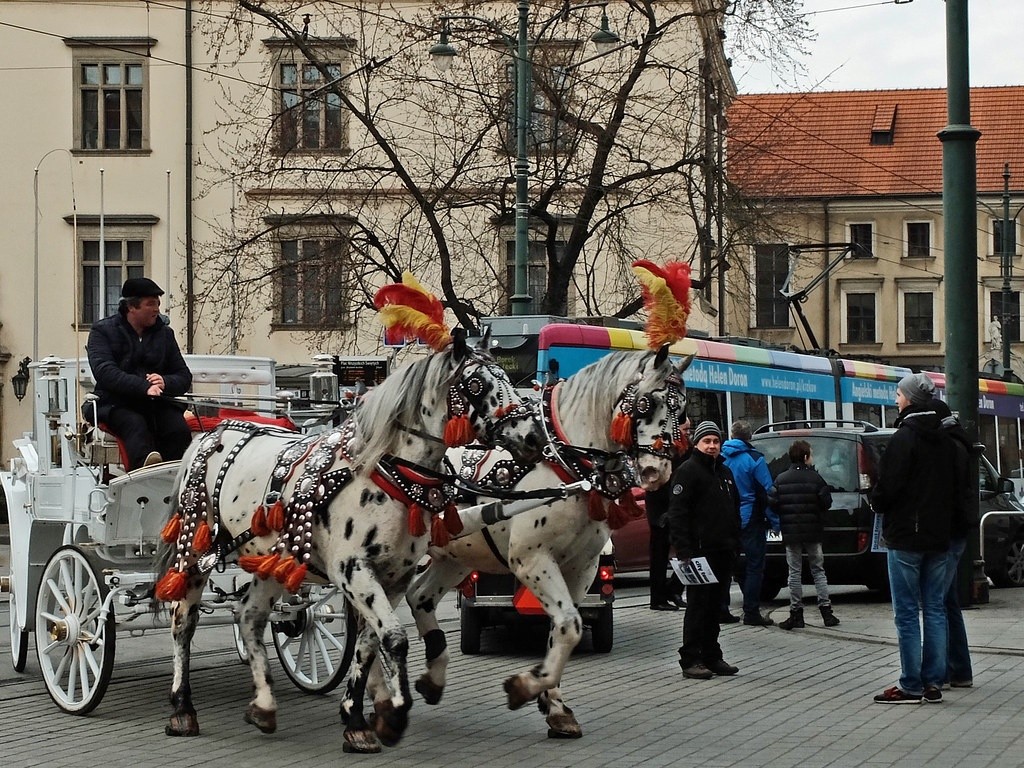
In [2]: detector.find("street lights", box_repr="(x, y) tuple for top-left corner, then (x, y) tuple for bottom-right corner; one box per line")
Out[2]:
(426, 1), (624, 324)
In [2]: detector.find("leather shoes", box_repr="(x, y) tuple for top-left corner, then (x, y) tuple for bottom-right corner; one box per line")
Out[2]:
(650, 602), (679, 611)
(669, 593), (687, 608)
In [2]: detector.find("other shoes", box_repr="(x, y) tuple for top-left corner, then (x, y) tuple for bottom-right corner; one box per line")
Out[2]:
(718, 613), (740, 623)
(743, 615), (774, 625)
(142, 451), (162, 467)
(951, 679), (972, 687)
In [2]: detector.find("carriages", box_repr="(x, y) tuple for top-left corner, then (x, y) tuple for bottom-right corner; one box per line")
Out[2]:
(0, 259), (695, 757)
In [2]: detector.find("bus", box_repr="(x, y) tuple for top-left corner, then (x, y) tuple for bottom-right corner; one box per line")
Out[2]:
(471, 241), (1024, 578)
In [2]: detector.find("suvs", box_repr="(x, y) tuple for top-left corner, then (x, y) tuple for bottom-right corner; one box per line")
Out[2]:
(734, 418), (1024, 601)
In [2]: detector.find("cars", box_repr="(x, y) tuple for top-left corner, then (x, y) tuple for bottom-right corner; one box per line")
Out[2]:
(454, 529), (617, 656)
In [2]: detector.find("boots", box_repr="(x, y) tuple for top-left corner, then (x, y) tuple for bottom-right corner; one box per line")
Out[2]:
(779, 607), (805, 630)
(819, 604), (840, 626)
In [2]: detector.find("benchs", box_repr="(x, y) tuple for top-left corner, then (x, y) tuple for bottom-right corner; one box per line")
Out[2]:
(84, 370), (294, 464)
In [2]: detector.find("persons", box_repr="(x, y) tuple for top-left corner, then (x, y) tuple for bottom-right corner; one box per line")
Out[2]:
(869, 373), (976, 705)
(767, 441), (841, 631)
(668, 421), (739, 679)
(933, 399), (978, 692)
(82, 279), (193, 474)
(720, 420), (780, 626)
(988, 316), (1003, 350)
(644, 417), (694, 611)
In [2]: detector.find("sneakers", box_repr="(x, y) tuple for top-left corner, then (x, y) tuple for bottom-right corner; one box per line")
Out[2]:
(874, 686), (923, 703)
(683, 663), (712, 680)
(705, 658), (739, 675)
(923, 690), (943, 702)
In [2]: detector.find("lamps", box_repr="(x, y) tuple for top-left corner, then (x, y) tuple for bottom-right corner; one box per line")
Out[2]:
(12, 356), (32, 402)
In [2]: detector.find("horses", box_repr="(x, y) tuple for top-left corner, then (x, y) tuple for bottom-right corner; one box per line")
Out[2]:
(164, 321), (549, 752)
(364, 344), (698, 748)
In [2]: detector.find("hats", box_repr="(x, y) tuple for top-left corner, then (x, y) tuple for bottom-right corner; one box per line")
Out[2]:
(897, 373), (935, 402)
(693, 421), (722, 445)
(122, 278), (165, 298)
(932, 399), (951, 420)
